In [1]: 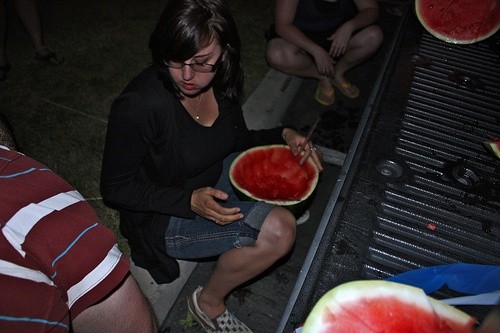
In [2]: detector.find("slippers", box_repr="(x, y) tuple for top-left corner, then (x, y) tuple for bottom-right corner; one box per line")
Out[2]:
(0, 64), (8, 81)
(316, 84), (334, 105)
(34, 51), (64, 65)
(333, 80), (359, 97)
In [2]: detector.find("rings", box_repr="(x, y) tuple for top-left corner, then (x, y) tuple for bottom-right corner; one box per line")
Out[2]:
(310, 146), (316, 151)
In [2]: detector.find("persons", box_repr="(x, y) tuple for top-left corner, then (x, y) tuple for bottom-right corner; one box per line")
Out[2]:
(100, 0), (324, 333)
(265, 0), (383, 107)
(0, 120), (156, 333)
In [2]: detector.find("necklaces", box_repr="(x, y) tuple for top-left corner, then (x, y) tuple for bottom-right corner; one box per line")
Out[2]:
(183, 91), (205, 122)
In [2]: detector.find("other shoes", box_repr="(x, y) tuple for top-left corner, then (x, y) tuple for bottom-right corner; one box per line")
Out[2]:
(188, 287), (251, 333)
(294, 208), (311, 225)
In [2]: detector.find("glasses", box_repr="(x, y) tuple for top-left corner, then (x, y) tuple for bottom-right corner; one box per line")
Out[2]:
(165, 50), (224, 74)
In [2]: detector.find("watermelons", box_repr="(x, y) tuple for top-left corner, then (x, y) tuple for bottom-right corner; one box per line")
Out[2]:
(300, 279), (478, 333)
(415, 0), (500, 44)
